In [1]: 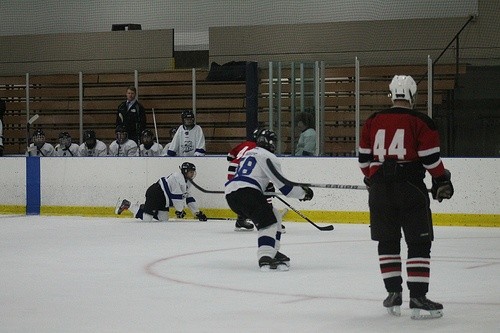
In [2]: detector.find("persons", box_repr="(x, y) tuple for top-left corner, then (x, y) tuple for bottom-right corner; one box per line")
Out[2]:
(78, 130), (107, 156)
(358, 75), (454, 320)
(226, 129), (286, 233)
(26, 130), (54, 156)
(115, 87), (146, 145)
(295, 112), (316, 156)
(54, 132), (79, 156)
(109, 126), (137, 156)
(115, 162), (207, 221)
(162, 127), (178, 155)
(0, 98), (7, 156)
(136, 129), (163, 156)
(166, 110), (207, 156)
(225, 129), (314, 271)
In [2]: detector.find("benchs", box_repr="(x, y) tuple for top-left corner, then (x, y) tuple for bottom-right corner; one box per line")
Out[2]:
(0, 64), (467, 156)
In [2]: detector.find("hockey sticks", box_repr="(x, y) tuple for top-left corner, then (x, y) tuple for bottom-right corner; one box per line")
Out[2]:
(193, 217), (250, 221)
(274, 195), (334, 231)
(266, 158), (437, 194)
(178, 166), (284, 196)
(151, 107), (160, 145)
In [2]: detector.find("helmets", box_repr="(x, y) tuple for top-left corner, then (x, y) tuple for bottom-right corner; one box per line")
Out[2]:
(248, 126), (259, 141)
(389, 74), (417, 104)
(83, 130), (96, 148)
(115, 125), (129, 144)
(31, 129), (45, 146)
(182, 162), (196, 180)
(141, 130), (154, 145)
(59, 132), (71, 150)
(256, 128), (276, 152)
(170, 128), (177, 139)
(181, 111), (194, 127)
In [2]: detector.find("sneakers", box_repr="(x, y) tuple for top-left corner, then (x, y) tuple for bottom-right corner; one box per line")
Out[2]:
(234, 218), (253, 232)
(281, 224), (285, 234)
(382, 287), (402, 316)
(115, 197), (129, 215)
(409, 290), (443, 319)
(259, 251), (290, 271)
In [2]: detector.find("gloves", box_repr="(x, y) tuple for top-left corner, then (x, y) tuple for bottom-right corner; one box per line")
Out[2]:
(175, 210), (185, 218)
(196, 211), (207, 221)
(364, 175), (372, 190)
(299, 185), (314, 202)
(431, 172), (453, 203)
